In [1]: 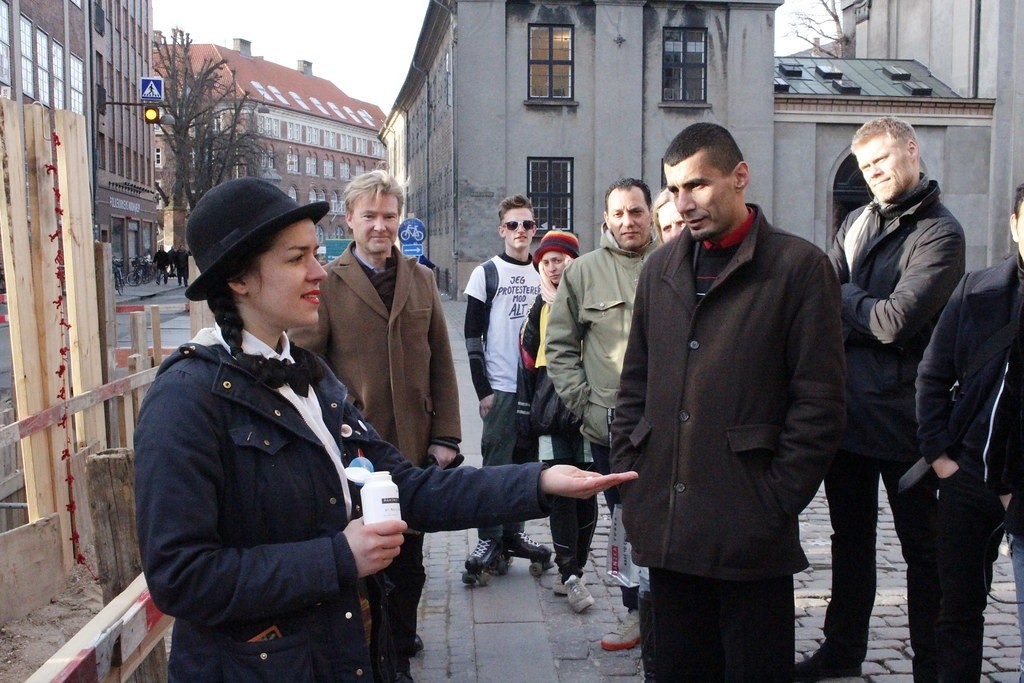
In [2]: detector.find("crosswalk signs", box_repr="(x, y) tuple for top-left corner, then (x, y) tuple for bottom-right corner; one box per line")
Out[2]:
(139, 77), (165, 102)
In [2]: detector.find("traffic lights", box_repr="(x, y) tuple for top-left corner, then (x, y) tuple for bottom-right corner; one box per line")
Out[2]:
(145, 106), (159, 123)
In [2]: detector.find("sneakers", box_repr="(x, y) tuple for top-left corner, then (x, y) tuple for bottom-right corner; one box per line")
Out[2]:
(552, 571), (567, 595)
(564, 577), (593, 614)
(600, 610), (644, 651)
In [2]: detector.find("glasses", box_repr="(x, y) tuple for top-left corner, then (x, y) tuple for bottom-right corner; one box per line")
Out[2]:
(502, 221), (536, 231)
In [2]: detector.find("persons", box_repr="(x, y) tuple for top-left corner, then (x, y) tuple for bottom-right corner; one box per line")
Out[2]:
(605, 123), (846, 683)
(144, 251), (151, 259)
(916, 187), (1023, 683)
(795, 116), (966, 683)
(653, 190), (686, 245)
(546, 179), (658, 650)
(132, 177), (640, 683)
(461, 192), (554, 586)
(516, 228), (598, 613)
(419, 255), (435, 269)
(285, 169), (461, 683)
(112, 256), (123, 273)
(152, 245), (192, 287)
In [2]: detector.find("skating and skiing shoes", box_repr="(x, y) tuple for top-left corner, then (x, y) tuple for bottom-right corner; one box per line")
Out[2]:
(461, 536), (503, 586)
(502, 529), (556, 576)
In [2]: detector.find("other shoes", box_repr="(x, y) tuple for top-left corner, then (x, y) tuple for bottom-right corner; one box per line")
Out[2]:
(414, 634), (423, 650)
(794, 644), (862, 683)
(157, 281), (160, 285)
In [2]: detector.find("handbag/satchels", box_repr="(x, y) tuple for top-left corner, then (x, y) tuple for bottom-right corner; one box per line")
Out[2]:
(606, 504), (640, 588)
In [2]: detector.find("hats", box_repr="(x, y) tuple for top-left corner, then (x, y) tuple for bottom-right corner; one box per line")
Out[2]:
(534, 229), (579, 263)
(185, 176), (329, 302)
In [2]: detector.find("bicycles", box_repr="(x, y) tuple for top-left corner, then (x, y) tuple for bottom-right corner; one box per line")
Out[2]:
(111, 254), (159, 297)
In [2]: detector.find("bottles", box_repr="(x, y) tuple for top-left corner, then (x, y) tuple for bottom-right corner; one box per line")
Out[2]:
(345, 466), (402, 535)
